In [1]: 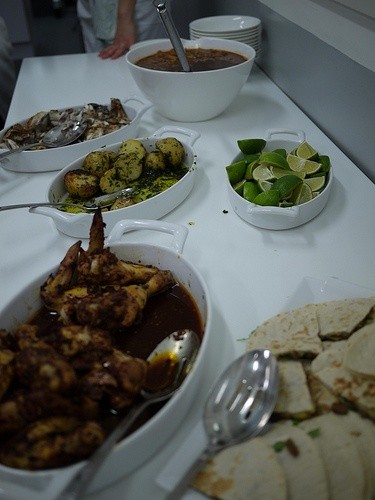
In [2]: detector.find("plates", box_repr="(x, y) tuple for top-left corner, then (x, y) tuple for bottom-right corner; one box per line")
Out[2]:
(189, 15), (264, 62)
(155, 272), (375, 500)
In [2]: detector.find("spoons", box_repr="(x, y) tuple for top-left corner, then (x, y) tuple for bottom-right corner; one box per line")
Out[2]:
(163, 350), (278, 499)
(0, 121), (89, 162)
(0, 187), (137, 212)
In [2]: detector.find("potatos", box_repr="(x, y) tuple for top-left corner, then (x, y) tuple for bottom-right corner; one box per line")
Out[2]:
(64, 137), (185, 211)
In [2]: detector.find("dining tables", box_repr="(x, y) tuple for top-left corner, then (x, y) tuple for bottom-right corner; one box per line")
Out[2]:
(0, 41), (375, 500)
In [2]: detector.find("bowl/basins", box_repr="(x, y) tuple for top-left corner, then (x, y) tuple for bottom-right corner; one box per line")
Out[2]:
(0, 94), (155, 173)
(226, 128), (333, 231)
(29, 124), (201, 240)
(125, 37), (257, 123)
(0, 217), (213, 500)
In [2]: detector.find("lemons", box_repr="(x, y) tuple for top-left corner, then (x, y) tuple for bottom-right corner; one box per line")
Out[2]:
(226, 138), (330, 208)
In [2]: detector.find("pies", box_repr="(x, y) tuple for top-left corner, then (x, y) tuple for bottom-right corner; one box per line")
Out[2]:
(193, 296), (375, 500)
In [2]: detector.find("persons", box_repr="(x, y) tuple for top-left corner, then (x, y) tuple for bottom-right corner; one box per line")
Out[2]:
(94, 0), (171, 60)
(77, 0), (105, 53)
